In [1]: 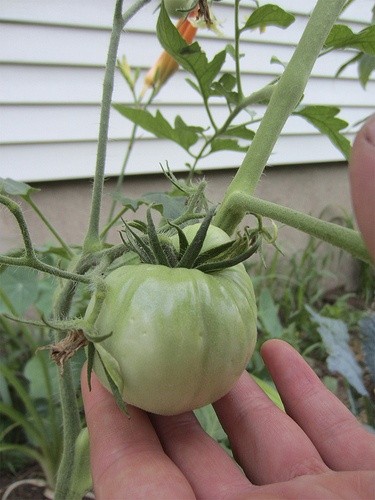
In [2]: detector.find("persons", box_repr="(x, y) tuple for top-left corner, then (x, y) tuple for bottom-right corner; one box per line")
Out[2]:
(78, 109), (375, 500)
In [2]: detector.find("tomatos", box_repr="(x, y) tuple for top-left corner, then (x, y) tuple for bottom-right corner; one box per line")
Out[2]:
(81, 224), (258, 418)
